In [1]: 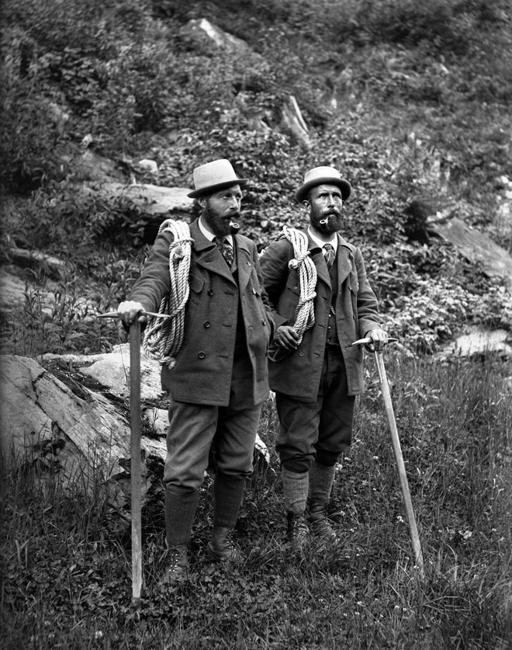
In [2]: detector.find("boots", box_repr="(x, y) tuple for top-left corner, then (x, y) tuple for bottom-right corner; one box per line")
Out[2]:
(208, 524), (244, 565)
(159, 546), (189, 586)
(308, 498), (336, 544)
(287, 511), (311, 546)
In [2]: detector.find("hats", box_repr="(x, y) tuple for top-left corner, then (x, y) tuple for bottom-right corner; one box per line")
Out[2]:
(188, 159), (249, 197)
(295, 166), (350, 203)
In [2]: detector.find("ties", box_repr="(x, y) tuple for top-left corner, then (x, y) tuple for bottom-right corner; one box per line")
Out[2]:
(323, 245), (336, 266)
(217, 239), (234, 269)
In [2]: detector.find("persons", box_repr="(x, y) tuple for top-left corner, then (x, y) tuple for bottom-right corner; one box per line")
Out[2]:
(118, 160), (275, 587)
(259, 167), (387, 543)
